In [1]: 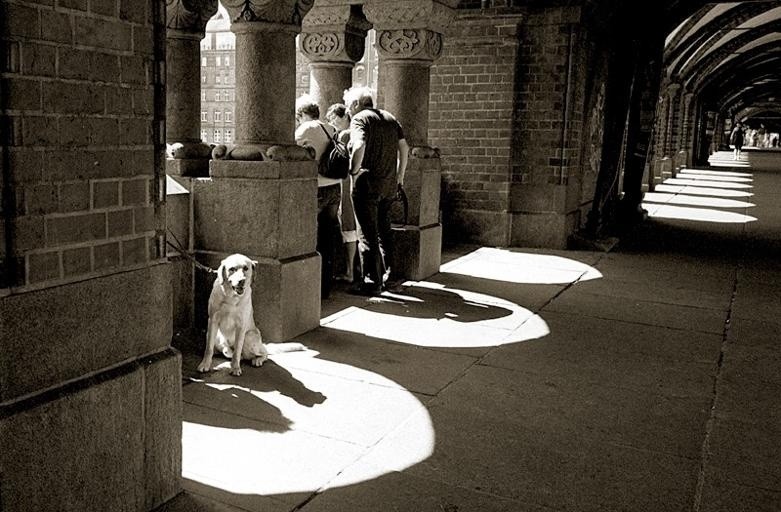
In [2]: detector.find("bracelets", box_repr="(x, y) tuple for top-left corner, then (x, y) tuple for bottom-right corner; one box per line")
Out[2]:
(349, 170), (359, 175)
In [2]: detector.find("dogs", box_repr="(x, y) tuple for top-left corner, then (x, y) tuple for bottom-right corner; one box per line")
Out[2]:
(197, 253), (307, 376)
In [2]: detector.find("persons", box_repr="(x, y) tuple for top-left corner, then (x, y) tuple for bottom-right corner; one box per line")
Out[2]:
(325, 103), (364, 283)
(729, 123), (744, 161)
(343, 88), (409, 296)
(744, 125), (778, 148)
(294, 104), (342, 300)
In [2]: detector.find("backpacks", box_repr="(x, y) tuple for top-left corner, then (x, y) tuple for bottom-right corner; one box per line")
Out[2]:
(312, 120), (349, 179)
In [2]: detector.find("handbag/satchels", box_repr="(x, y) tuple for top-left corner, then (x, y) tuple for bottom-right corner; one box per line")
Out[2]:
(389, 183), (408, 228)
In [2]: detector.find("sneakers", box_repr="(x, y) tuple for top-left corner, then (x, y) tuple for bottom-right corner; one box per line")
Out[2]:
(381, 277), (404, 293)
(343, 276), (382, 297)
